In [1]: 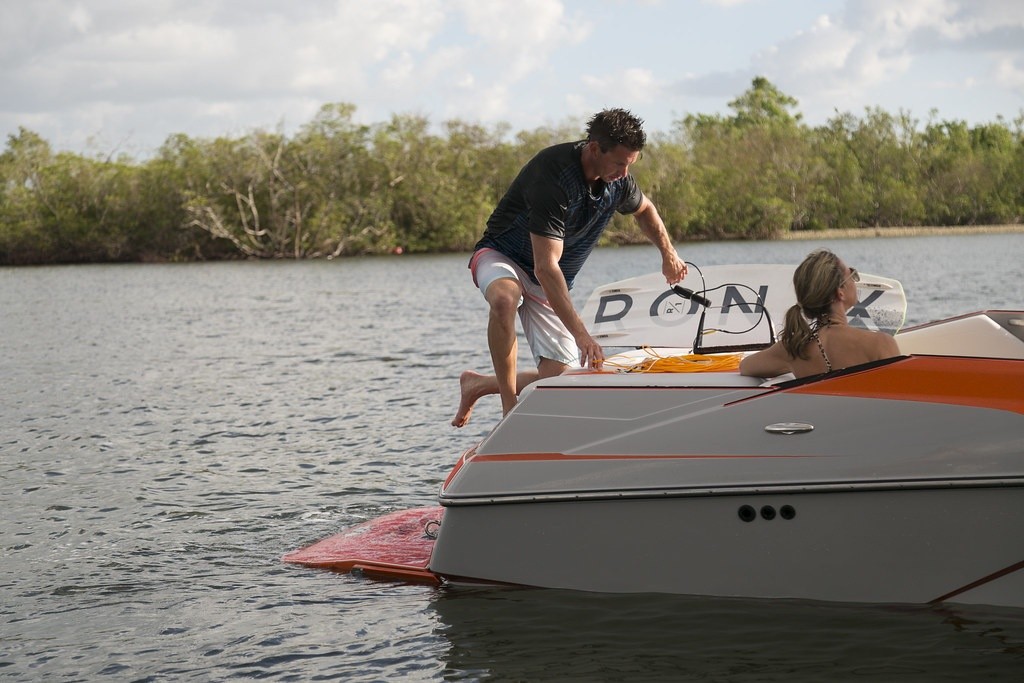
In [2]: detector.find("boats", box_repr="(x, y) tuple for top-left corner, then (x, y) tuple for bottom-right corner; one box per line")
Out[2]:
(427, 261), (1024, 608)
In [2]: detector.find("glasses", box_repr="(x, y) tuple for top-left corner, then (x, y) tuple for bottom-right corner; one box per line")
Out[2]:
(840, 266), (864, 288)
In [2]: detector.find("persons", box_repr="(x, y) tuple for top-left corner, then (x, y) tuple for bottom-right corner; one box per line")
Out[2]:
(739, 249), (900, 380)
(452, 106), (689, 429)
